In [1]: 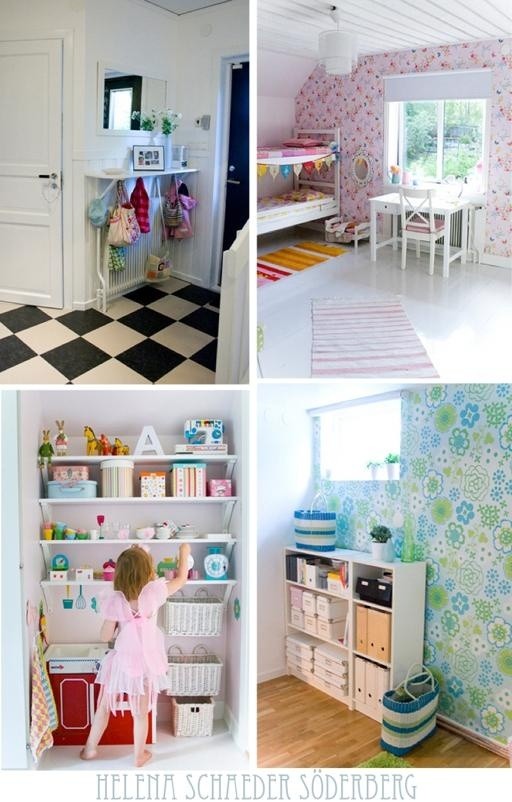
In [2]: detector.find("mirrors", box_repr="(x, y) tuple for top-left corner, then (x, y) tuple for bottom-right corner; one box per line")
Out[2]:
(350, 145), (375, 188)
(96, 59), (172, 139)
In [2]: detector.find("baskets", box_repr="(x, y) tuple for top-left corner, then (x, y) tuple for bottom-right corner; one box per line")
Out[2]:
(164, 646), (223, 696)
(163, 587), (225, 636)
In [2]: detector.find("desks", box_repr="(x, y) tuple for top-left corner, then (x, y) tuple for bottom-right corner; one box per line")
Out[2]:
(368, 188), (470, 278)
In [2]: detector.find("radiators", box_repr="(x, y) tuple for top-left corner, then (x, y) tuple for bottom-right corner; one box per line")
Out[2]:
(105, 196), (170, 297)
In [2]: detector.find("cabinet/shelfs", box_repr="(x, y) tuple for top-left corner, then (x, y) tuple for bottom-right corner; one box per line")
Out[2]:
(85, 162), (200, 312)
(18, 391), (258, 769)
(284, 545), (428, 723)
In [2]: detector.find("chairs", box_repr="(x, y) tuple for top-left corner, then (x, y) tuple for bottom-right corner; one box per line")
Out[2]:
(398, 185), (445, 275)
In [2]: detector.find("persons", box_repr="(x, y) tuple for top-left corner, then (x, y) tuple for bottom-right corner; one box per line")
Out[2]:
(36, 429), (55, 470)
(79, 544), (191, 767)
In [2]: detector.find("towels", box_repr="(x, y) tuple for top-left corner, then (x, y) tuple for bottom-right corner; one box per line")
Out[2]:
(29, 650), (53, 763)
(38, 655), (59, 733)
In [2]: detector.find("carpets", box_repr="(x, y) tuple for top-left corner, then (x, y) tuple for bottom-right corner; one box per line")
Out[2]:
(352, 749), (416, 768)
(257, 239), (353, 290)
(311, 296), (439, 379)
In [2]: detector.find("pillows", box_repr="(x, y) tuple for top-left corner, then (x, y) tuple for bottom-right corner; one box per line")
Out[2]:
(282, 138), (322, 148)
(280, 189), (324, 202)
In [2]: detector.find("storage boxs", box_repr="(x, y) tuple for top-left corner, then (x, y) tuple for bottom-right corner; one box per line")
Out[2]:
(100, 460), (135, 498)
(205, 478), (233, 497)
(367, 607), (377, 659)
(357, 577), (391, 607)
(316, 616), (346, 639)
(168, 462), (207, 498)
(286, 650), (313, 672)
(286, 663), (312, 685)
(50, 465), (90, 481)
(302, 591), (317, 617)
(365, 660), (376, 709)
(48, 480), (98, 499)
(285, 633), (313, 661)
(356, 604), (367, 653)
(290, 607), (304, 628)
(164, 653), (224, 697)
(316, 593), (347, 620)
(43, 643), (156, 745)
(164, 594), (226, 637)
(311, 675), (348, 703)
(312, 665), (347, 688)
(289, 586), (302, 608)
(138, 470), (166, 498)
(376, 665), (390, 713)
(303, 613), (317, 634)
(377, 610), (391, 663)
(354, 656), (365, 704)
(312, 643), (348, 679)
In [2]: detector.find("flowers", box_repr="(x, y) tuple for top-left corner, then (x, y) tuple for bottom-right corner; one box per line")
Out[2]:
(367, 460), (381, 467)
(158, 105), (184, 138)
(385, 453), (400, 463)
(369, 525), (393, 542)
(131, 107), (159, 132)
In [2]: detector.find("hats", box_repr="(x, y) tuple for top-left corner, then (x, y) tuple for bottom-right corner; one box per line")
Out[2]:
(88, 199), (108, 227)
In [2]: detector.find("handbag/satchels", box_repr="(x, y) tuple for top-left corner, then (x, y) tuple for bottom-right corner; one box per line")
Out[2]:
(108, 180), (141, 247)
(161, 174), (184, 227)
(380, 664), (440, 757)
(294, 493), (337, 551)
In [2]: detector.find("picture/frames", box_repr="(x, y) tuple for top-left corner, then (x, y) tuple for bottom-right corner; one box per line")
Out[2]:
(132, 144), (165, 172)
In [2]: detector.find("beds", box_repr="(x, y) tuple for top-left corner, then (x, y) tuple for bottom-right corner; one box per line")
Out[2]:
(324, 215), (371, 249)
(257, 129), (341, 232)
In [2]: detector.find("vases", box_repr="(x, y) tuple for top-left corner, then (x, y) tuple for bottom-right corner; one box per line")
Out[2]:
(371, 541), (395, 562)
(370, 464), (380, 480)
(163, 137), (173, 170)
(386, 464), (399, 480)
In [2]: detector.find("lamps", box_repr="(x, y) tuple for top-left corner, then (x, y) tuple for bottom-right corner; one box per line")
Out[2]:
(315, 19), (360, 77)
